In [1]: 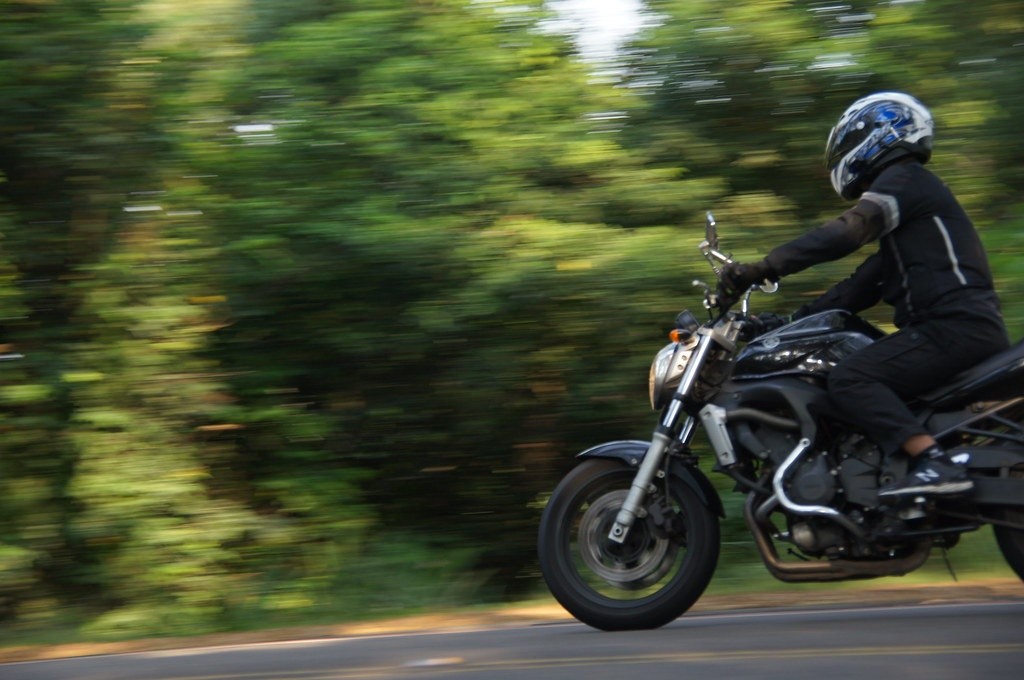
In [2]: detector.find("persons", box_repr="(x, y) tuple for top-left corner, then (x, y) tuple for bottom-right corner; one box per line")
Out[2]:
(719, 91), (1010, 495)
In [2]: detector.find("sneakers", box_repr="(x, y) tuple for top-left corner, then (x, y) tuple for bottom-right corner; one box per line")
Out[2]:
(877, 456), (973, 496)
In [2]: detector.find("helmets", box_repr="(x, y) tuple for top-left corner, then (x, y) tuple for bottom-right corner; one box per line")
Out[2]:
(825, 90), (935, 202)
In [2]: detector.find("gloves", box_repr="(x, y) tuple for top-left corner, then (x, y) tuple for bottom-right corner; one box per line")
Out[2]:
(750, 312), (790, 342)
(713, 259), (755, 306)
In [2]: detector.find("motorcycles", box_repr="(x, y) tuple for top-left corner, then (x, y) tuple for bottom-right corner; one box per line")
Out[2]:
(538, 207), (1024, 632)
